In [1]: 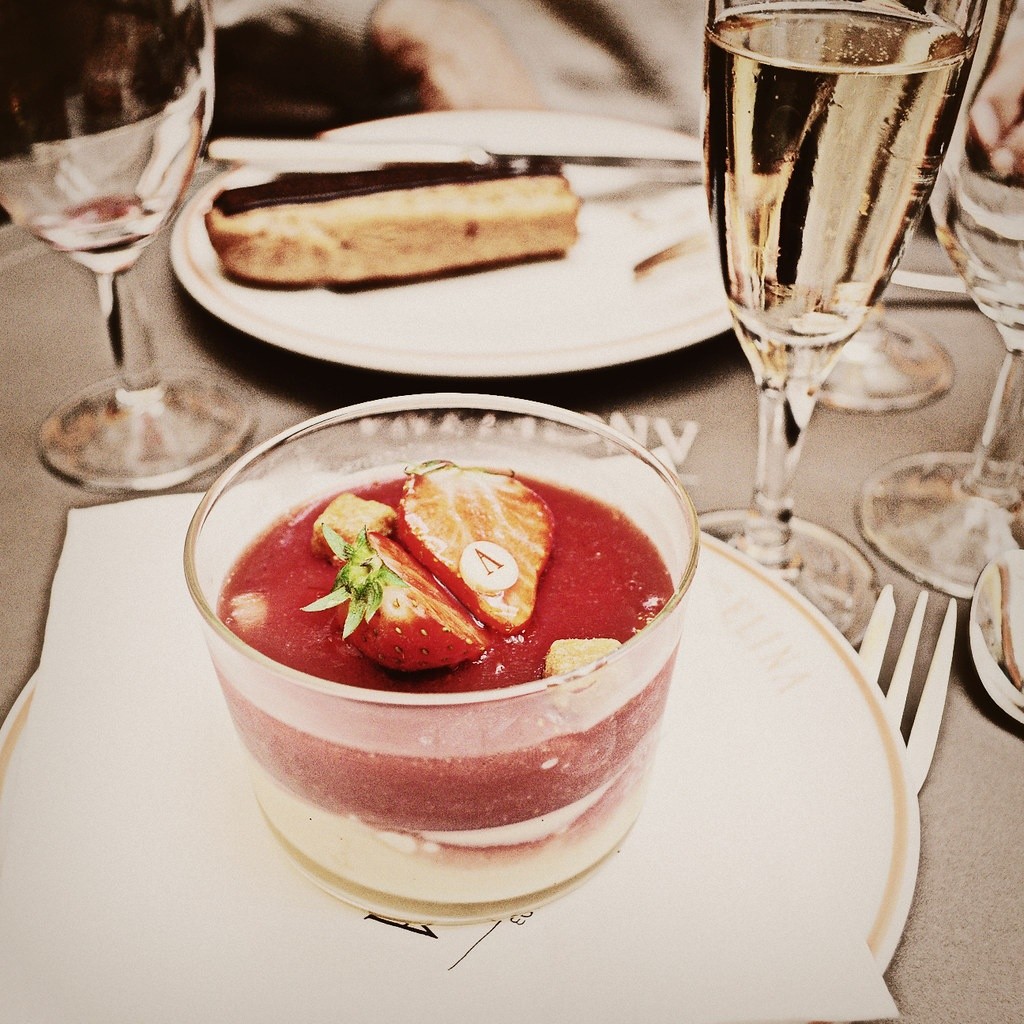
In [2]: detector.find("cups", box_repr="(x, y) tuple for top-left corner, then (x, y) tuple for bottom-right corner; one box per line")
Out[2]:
(182, 393), (703, 922)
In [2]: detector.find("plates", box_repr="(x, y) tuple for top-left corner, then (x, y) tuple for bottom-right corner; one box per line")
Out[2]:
(2, 519), (926, 1022)
(170, 110), (780, 380)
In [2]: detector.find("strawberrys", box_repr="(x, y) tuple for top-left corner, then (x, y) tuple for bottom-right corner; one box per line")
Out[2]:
(295, 518), (493, 671)
(398, 468), (555, 634)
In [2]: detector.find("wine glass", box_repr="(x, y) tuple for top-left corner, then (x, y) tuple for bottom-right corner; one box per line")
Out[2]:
(676, 1), (993, 648)
(853, 2), (1024, 591)
(1, 0), (262, 490)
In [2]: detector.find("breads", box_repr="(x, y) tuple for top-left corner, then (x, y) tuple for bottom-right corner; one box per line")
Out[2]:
(204, 174), (581, 287)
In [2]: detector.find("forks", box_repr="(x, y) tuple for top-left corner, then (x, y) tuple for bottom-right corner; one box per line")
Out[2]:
(845, 587), (959, 795)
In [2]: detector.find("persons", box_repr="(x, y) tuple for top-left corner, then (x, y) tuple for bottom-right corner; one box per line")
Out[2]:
(80, 1), (1024, 185)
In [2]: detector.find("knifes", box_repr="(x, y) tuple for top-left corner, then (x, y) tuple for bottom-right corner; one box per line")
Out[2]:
(212, 155), (706, 213)
